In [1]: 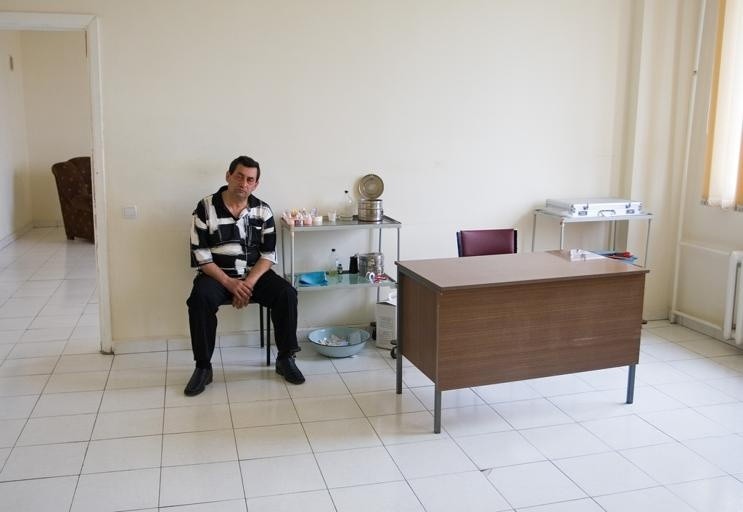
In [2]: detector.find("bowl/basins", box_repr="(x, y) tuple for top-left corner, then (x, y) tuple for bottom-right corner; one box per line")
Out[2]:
(359, 252), (385, 277)
(308, 326), (370, 358)
(358, 198), (383, 222)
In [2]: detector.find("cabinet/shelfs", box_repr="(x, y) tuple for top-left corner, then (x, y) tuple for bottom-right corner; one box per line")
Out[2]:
(277, 213), (401, 359)
(531, 207), (655, 329)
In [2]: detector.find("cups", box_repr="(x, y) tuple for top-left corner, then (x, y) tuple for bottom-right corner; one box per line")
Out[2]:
(328, 209), (337, 222)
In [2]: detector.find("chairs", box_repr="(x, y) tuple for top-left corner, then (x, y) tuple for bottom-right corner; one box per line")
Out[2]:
(189, 250), (272, 368)
(456, 229), (517, 258)
(51, 156), (93, 245)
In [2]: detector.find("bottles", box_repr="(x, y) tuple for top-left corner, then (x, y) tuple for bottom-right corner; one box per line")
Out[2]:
(340, 190), (353, 221)
(328, 248), (337, 277)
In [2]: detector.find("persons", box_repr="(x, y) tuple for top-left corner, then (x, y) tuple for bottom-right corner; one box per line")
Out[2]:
(182, 154), (306, 396)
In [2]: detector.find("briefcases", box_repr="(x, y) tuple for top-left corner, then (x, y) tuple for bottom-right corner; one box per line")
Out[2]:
(546, 197), (642, 218)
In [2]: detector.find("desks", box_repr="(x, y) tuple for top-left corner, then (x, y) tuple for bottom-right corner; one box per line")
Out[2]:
(395, 250), (649, 435)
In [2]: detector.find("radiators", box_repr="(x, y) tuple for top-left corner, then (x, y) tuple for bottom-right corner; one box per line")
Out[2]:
(723, 250), (743, 348)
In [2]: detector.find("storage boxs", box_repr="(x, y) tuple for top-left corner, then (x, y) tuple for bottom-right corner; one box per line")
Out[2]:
(374, 296), (397, 349)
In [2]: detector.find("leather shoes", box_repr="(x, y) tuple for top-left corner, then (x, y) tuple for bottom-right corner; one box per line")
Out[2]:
(183, 362), (213, 395)
(275, 358), (306, 385)
